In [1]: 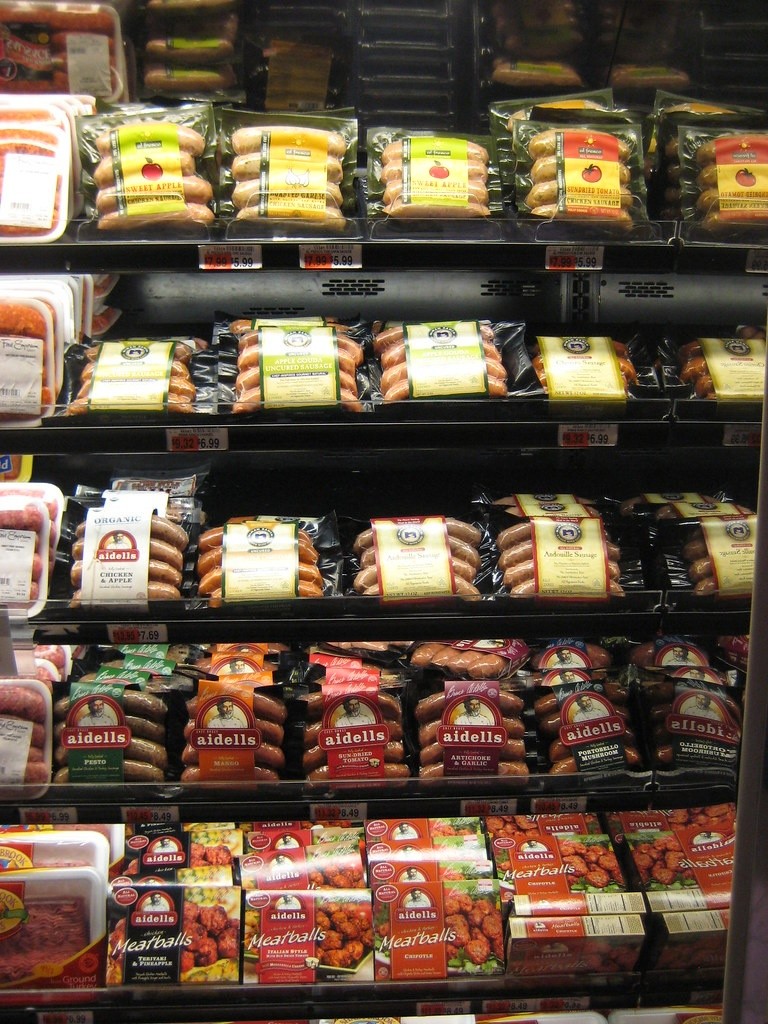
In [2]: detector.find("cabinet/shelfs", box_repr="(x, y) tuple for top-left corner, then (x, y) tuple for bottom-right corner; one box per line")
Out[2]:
(0, 218), (768, 1024)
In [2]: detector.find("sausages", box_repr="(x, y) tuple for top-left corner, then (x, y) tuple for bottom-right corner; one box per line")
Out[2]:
(1, 1), (768, 795)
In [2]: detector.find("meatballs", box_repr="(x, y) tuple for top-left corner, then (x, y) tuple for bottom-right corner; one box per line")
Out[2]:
(111, 802), (739, 978)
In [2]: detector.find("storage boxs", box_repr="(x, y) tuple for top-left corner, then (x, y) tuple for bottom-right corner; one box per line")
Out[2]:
(124, 828), (244, 882)
(370, 878), (505, 982)
(245, 826), (364, 853)
(647, 908), (729, 970)
(365, 857), (494, 893)
(490, 832), (626, 914)
(362, 834), (489, 865)
(105, 883), (243, 984)
(107, 865), (233, 885)
(506, 892), (647, 924)
(0, 831), (110, 888)
(644, 886), (731, 939)
(236, 839), (365, 891)
(506, 914), (646, 977)
(483, 811), (604, 835)
(621, 821), (733, 892)
(0, 823), (126, 881)
(243, 888), (375, 983)
(610, 802), (736, 840)
(363, 818), (481, 841)
(0, 865), (107, 990)
(0, 0), (129, 623)
